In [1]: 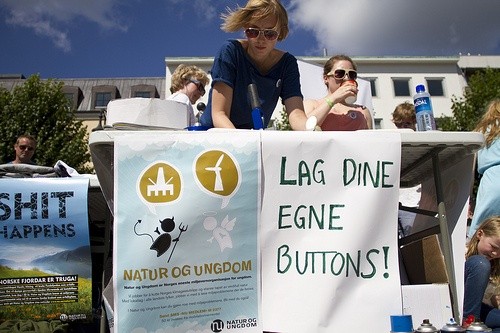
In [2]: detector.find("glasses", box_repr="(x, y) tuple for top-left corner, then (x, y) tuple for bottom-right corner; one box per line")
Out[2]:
(395, 122), (414, 128)
(327, 69), (357, 80)
(244, 28), (280, 41)
(17, 145), (35, 151)
(189, 79), (205, 96)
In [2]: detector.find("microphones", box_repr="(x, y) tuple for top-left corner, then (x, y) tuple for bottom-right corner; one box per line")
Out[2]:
(247, 83), (267, 130)
(197, 103), (206, 122)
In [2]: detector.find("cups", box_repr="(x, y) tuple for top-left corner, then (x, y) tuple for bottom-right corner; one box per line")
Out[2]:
(340, 80), (358, 105)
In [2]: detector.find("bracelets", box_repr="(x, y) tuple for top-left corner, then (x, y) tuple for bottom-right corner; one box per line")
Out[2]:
(325, 98), (335, 109)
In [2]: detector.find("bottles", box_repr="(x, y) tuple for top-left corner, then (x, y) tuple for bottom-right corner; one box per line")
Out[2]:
(413, 85), (437, 133)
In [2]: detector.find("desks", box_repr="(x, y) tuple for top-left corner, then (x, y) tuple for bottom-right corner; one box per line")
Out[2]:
(88, 129), (486, 332)
(0, 174), (110, 333)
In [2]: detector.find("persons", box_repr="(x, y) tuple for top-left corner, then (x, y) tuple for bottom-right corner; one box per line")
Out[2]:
(302, 54), (373, 131)
(390, 102), (417, 131)
(4, 134), (39, 165)
(468, 100), (500, 238)
(163, 63), (210, 126)
(463, 217), (500, 333)
(199, 0), (322, 131)
